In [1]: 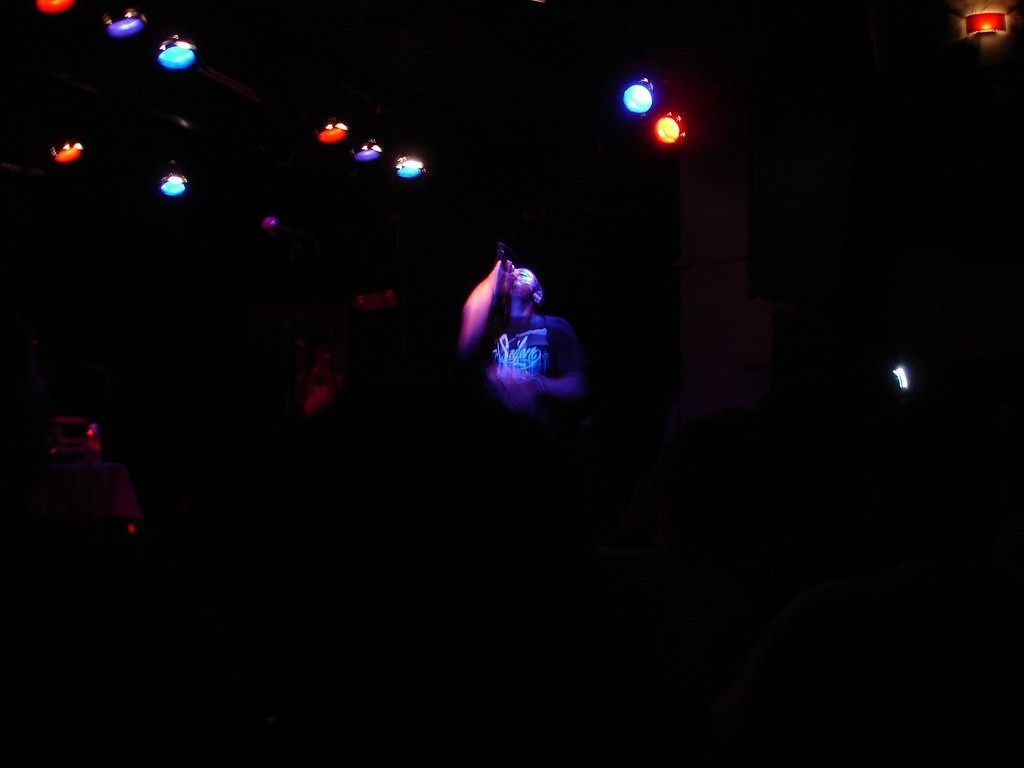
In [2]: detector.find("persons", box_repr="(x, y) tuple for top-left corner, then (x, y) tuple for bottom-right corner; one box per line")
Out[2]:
(590, 416), (1024, 736)
(454, 255), (591, 435)
(299, 331), (345, 417)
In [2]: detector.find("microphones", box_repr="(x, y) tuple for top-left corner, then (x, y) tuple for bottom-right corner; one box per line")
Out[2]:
(498, 243), (507, 271)
